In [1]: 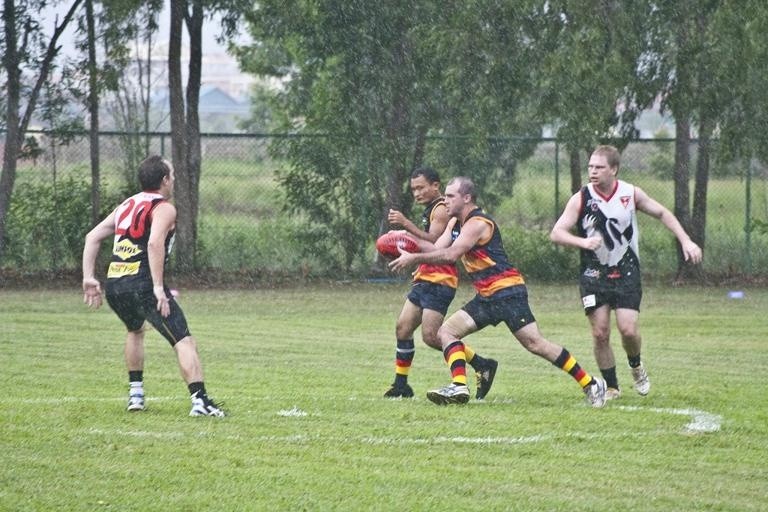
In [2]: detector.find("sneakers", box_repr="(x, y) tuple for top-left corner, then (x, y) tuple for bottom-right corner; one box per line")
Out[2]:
(583, 376), (607, 408)
(631, 360), (651, 396)
(425, 385), (468, 405)
(384, 385), (414, 398)
(608, 387), (621, 403)
(124, 394), (143, 412)
(190, 404), (226, 416)
(475, 360), (499, 401)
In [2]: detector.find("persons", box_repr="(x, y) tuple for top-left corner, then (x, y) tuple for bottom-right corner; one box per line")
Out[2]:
(387, 176), (609, 410)
(81, 155), (231, 418)
(548, 145), (704, 402)
(382, 166), (500, 401)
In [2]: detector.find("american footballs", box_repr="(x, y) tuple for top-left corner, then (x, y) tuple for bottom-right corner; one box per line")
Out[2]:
(375, 233), (422, 260)
